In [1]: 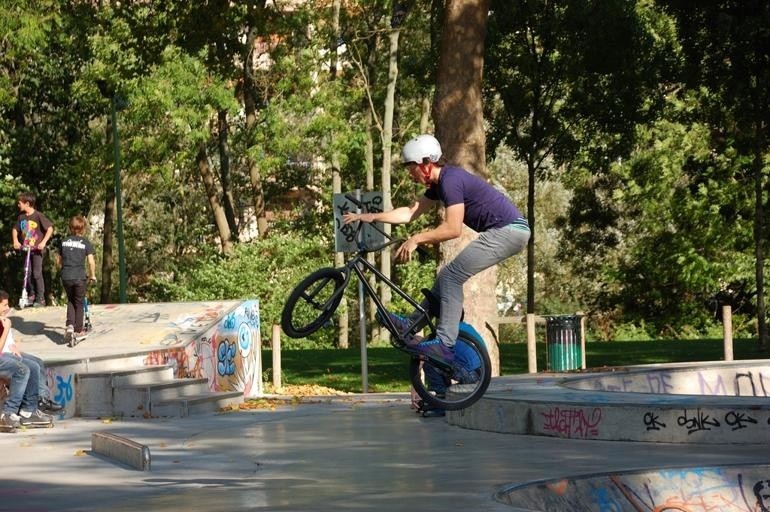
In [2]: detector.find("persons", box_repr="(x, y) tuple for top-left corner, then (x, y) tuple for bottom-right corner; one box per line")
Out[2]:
(409, 307), (489, 418)
(0, 289), (53, 429)
(55, 215), (97, 342)
(12, 190), (54, 308)
(343, 133), (532, 369)
(0, 324), (62, 413)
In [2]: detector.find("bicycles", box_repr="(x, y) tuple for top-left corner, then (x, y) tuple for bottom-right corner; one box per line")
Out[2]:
(281, 194), (491, 410)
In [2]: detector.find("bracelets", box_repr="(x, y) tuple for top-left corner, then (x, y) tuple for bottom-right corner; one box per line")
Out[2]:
(368, 212), (375, 222)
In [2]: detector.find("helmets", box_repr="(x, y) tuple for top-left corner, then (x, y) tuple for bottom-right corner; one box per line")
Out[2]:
(400, 134), (442, 165)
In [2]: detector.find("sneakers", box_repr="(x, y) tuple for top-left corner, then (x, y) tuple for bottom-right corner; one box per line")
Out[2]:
(64, 324), (86, 341)
(376, 308), (410, 334)
(28, 295), (46, 307)
(410, 335), (456, 366)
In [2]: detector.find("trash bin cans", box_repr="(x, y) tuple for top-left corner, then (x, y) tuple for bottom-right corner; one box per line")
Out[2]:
(538, 313), (589, 374)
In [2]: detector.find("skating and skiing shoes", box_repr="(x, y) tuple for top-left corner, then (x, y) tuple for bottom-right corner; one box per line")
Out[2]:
(18, 408), (54, 428)
(37, 397), (67, 415)
(418, 395), (445, 417)
(0, 413), (20, 432)
